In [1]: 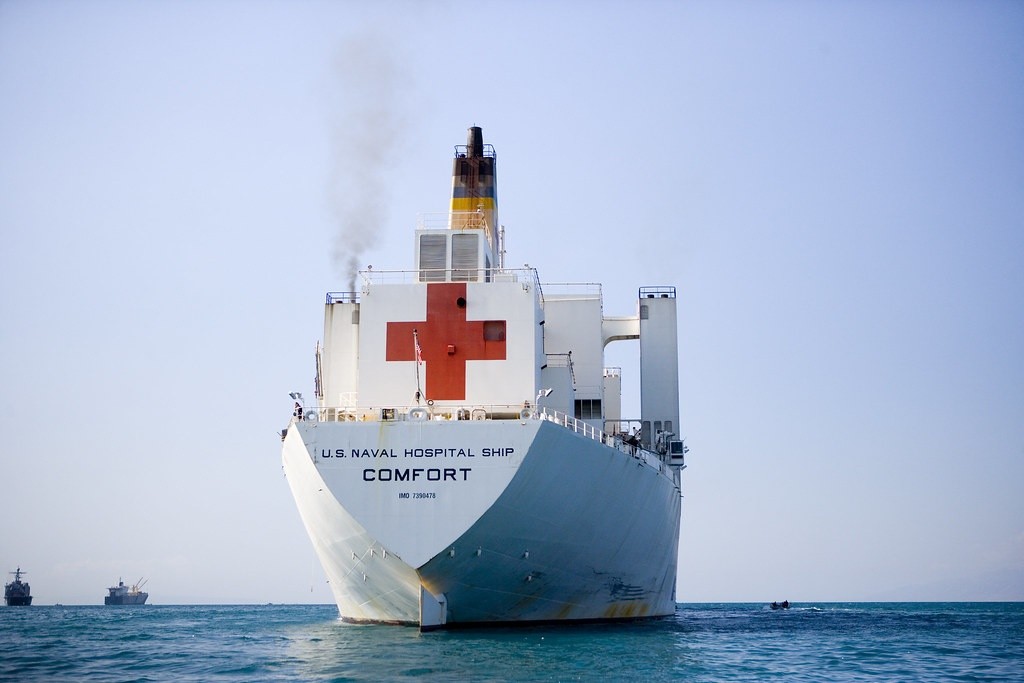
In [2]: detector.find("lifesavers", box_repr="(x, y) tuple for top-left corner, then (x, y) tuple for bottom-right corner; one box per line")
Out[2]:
(520, 408), (534, 420)
(305, 410), (319, 422)
(408, 407), (429, 422)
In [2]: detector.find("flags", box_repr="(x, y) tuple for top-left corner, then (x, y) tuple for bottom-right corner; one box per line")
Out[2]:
(415, 336), (422, 365)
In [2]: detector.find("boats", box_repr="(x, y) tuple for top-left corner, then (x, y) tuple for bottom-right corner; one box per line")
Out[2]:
(104, 575), (150, 606)
(3, 564), (34, 607)
(277, 125), (690, 632)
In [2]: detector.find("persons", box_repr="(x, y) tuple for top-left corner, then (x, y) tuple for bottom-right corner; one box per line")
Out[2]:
(628, 435), (642, 456)
(295, 402), (302, 419)
